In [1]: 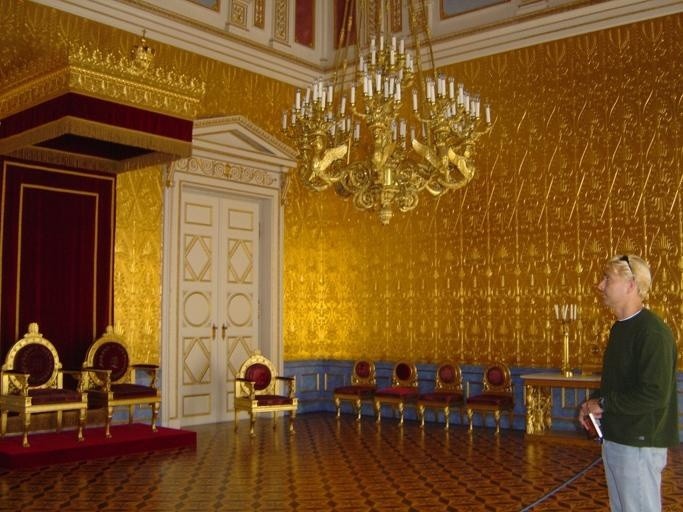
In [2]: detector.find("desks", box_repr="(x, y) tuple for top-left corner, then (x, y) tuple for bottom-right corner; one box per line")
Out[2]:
(520, 372), (602, 448)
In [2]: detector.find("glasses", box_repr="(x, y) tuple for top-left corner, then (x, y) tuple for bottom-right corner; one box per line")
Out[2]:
(619, 252), (635, 279)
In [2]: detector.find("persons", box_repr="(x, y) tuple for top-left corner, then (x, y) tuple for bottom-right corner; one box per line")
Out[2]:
(578, 255), (676, 512)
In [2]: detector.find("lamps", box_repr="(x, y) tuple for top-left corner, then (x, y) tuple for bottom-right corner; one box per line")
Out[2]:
(280, 0), (493, 225)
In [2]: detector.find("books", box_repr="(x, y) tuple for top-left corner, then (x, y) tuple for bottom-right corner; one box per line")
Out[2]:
(583, 413), (603, 440)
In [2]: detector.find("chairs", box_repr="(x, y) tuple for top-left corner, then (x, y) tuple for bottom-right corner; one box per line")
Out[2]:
(235, 350), (300, 438)
(81, 324), (162, 438)
(0, 322), (89, 448)
(333, 358), (516, 436)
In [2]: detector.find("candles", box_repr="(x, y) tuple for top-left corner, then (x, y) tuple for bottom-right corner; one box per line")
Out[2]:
(554, 303), (577, 321)
